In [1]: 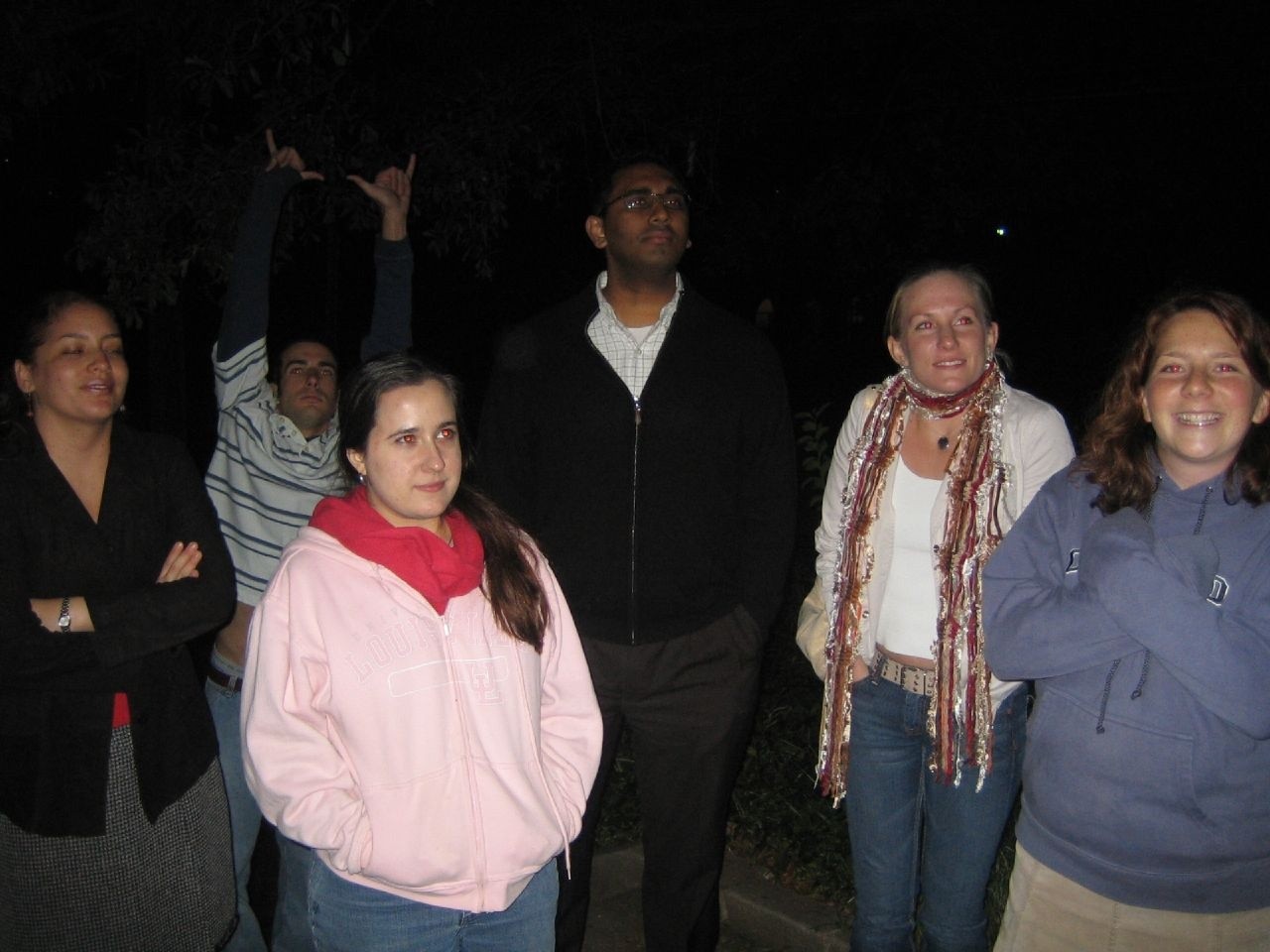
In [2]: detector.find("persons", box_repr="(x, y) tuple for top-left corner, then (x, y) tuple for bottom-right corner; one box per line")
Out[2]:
(0, 282), (237, 952)
(206, 125), (416, 952)
(482, 148), (810, 952)
(984, 299), (1270, 952)
(241, 356), (602, 952)
(798, 266), (1076, 952)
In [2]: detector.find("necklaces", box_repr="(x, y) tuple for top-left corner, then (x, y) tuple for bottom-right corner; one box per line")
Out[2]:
(921, 420), (961, 451)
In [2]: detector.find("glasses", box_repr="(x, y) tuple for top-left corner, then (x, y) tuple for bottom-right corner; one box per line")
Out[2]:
(602, 190), (690, 214)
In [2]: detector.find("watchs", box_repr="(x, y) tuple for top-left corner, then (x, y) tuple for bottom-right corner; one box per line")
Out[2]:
(58, 597), (72, 635)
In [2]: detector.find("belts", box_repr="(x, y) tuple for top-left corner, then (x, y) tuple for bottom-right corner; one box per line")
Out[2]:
(208, 665), (243, 692)
(867, 651), (935, 699)
